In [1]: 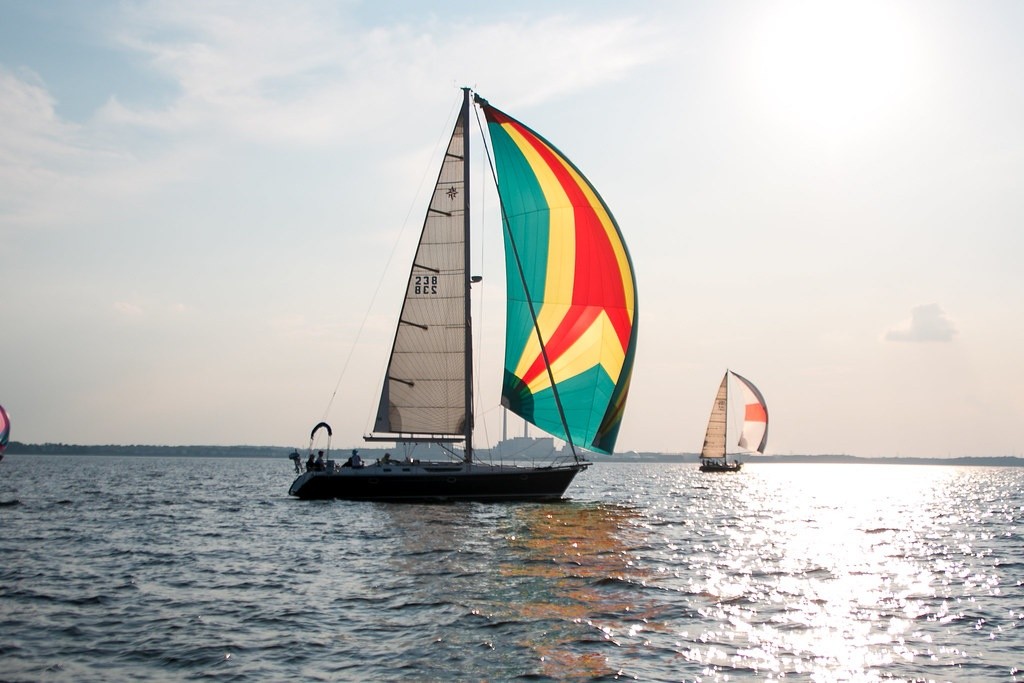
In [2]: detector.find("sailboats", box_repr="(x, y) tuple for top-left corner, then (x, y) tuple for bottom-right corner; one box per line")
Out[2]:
(698, 368), (769, 472)
(289, 87), (638, 506)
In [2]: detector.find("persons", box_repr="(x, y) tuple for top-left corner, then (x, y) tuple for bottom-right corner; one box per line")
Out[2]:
(289, 447), (399, 472)
